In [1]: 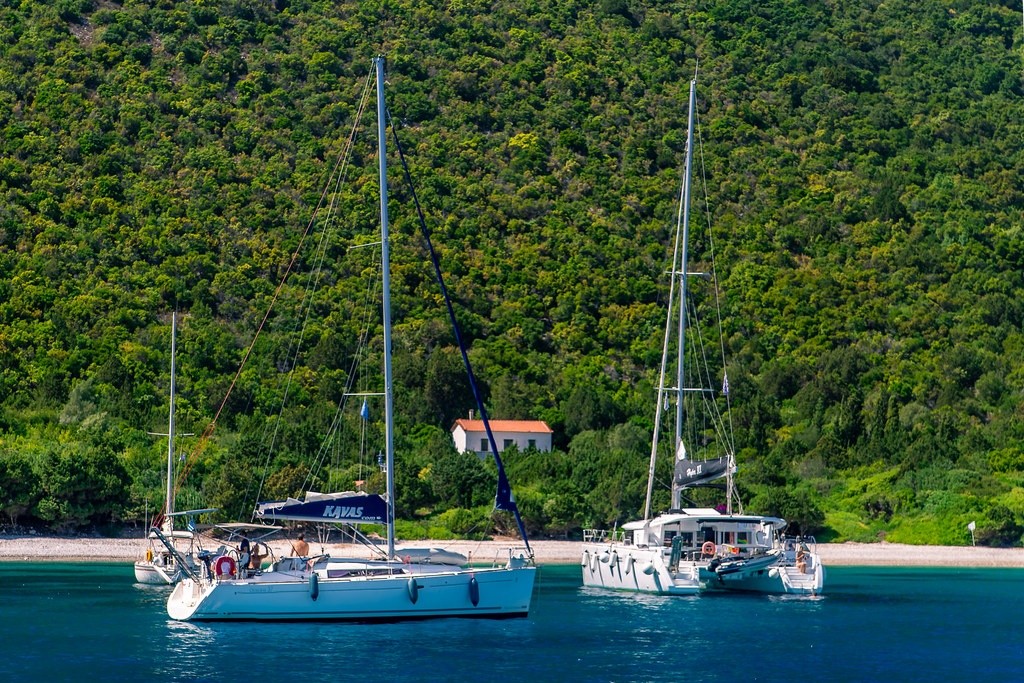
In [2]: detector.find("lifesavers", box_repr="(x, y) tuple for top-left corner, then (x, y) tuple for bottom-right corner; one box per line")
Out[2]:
(702, 541), (716, 555)
(215, 556), (236, 577)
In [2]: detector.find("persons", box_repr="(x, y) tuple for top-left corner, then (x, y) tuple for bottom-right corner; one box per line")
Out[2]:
(249, 544), (269, 571)
(795, 546), (806, 573)
(290, 532), (309, 556)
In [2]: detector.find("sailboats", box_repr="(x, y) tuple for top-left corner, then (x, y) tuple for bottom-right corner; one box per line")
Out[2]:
(582, 54), (824, 595)
(135, 53), (539, 621)
